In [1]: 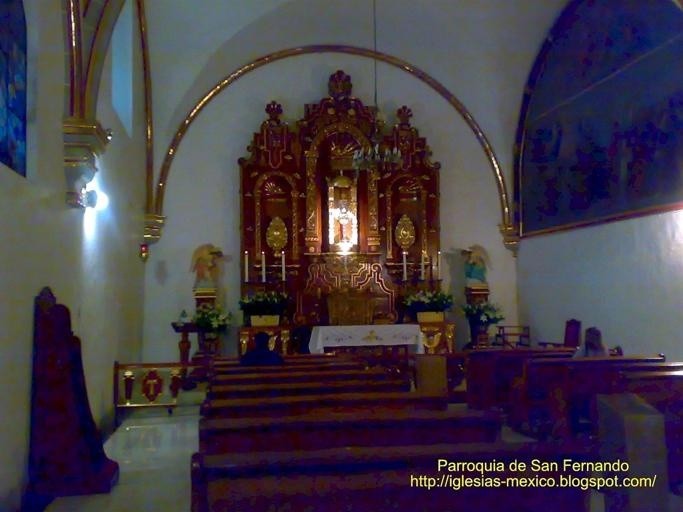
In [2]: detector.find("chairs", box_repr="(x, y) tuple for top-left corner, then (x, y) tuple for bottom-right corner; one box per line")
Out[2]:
(538, 318), (623, 356)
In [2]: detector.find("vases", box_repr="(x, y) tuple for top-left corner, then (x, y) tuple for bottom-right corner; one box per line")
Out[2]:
(205, 336), (221, 356)
(238, 290), (289, 315)
(460, 300), (505, 323)
(479, 323), (487, 334)
(416, 312), (445, 322)
(250, 315), (280, 328)
(194, 304), (233, 339)
(402, 290), (454, 312)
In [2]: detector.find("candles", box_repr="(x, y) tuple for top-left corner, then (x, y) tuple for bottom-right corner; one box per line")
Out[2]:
(403, 251), (441, 281)
(244, 250), (286, 282)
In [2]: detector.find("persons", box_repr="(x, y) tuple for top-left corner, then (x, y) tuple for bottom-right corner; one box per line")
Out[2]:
(239, 331), (285, 366)
(571, 326), (610, 358)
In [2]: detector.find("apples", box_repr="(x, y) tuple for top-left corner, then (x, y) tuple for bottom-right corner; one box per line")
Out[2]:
(244, 250), (286, 282)
(403, 251), (441, 281)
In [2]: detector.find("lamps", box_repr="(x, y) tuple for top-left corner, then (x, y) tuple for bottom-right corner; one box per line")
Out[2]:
(538, 318), (623, 356)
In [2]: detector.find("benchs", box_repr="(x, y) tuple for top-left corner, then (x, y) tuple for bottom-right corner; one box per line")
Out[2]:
(190, 348), (682, 512)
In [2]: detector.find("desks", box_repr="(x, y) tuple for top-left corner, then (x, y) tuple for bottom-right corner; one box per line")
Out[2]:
(313, 323), (420, 358)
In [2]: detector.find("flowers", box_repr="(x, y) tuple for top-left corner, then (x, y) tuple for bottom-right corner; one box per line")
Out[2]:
(460, 300), (505, 323)
(238, 290), (289, 315)
(194, 304), (233, 339)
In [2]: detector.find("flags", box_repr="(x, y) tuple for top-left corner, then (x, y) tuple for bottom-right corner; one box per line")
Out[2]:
(205, 336), (221, 356)
(402, 290), (454, 312)
(479, 323), (487, 334)
(416, 312), (445, 322)
(250, 315), (280, 328)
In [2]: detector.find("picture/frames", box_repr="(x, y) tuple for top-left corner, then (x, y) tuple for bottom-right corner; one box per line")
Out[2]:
(313, 323), (420, 358)
